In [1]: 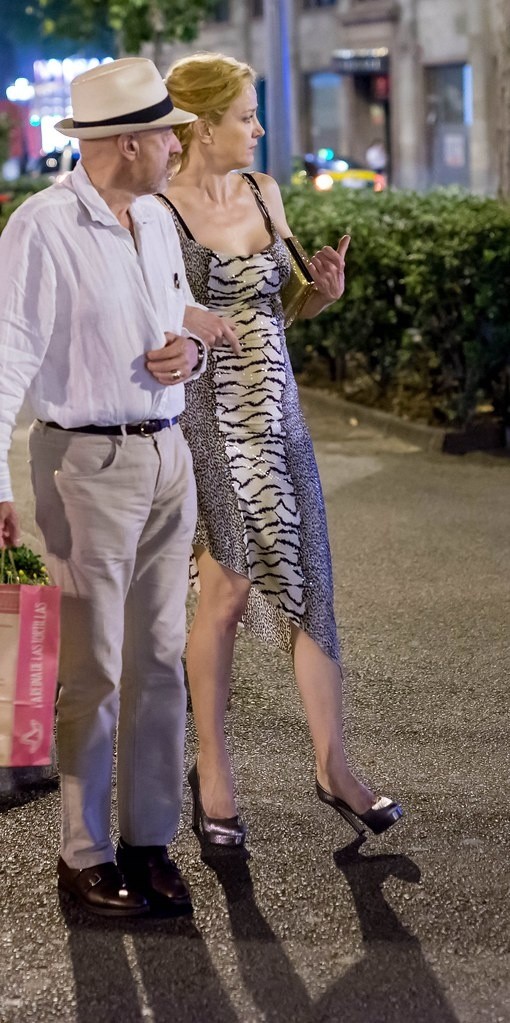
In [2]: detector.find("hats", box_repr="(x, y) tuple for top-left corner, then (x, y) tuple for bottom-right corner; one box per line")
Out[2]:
(53, 57), (198, 138)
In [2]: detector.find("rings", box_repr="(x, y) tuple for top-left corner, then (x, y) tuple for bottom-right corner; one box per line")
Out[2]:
(170, 368), (182, 381)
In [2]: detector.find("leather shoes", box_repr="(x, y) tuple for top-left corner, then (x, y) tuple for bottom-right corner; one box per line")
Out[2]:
(113, 837), (195, 912)
(56, 854), (149, 916)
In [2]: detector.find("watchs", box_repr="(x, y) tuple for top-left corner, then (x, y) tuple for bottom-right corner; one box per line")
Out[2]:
(186, 335), (206, 373)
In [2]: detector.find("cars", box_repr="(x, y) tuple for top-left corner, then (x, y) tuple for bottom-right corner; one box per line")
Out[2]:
(290, 161), (385, 193)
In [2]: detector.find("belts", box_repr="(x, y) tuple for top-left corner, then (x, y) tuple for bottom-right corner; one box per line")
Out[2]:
(36, 414), (181, 435)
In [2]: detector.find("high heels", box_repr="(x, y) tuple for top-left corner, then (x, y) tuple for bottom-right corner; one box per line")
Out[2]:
(188, 760), (246, 847)
(314, 772), (403, 835)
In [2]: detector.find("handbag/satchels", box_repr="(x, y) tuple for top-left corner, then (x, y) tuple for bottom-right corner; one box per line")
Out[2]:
(0, 543), (62, 766)
(281, 236), (317, 329)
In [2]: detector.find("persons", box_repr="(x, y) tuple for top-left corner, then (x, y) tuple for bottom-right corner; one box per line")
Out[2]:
(0, 57), (207, 922)
(145, 56), (405, 850)
